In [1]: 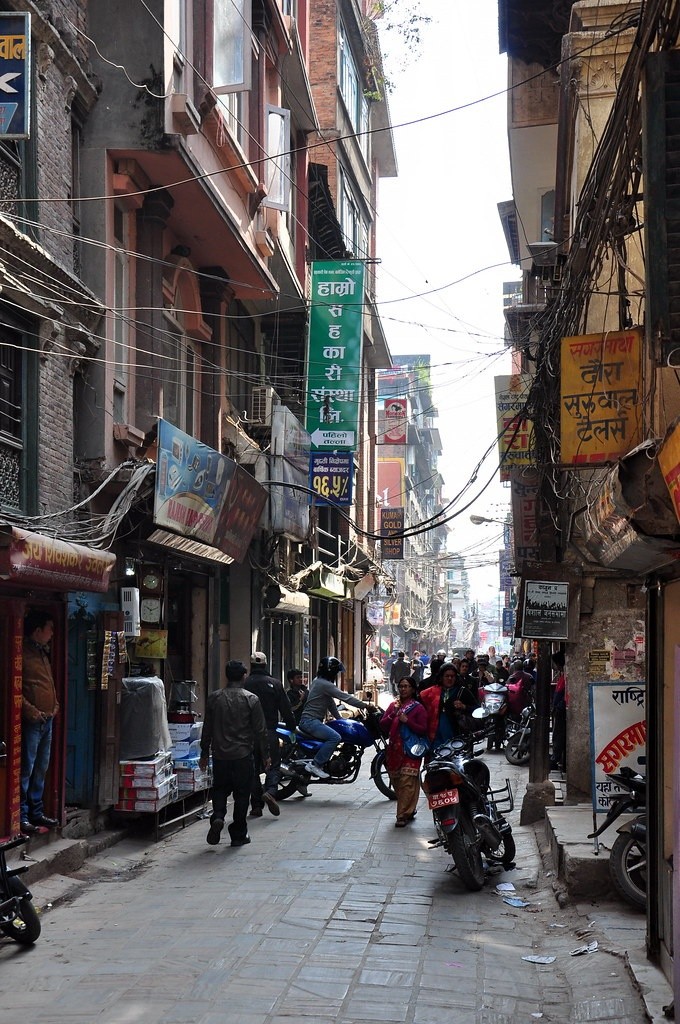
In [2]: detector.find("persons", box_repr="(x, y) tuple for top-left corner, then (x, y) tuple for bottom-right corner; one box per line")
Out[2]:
(21, 614), (61, 834)
(243, 651), (298, 817)
(294, 657), (375, 797)
(370, 646), (565, 826)
(199, 660), (273, 847)
(285, 669), (311, 726)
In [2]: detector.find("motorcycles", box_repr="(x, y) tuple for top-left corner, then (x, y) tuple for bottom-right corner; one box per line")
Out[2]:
(255, 692), (398, 802)
(410, 707), (516, 891)
(478, 676), (535, 765)
(586, 766), (647, 914)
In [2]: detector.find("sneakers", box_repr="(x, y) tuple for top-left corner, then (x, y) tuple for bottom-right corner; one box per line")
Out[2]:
(297, 785), (312, 797)
(29, 815), (59, 827)
(305, 761), (329, 778)
(20, 821), (39, 833)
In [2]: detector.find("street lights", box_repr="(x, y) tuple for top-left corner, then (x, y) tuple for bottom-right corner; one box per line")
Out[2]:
(488, 584), (501, 656)
(425, 589), (459, 658)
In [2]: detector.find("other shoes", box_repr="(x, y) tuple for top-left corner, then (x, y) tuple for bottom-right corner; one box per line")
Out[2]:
(232, 834), (251, 846)
(207, 818), (224, 845)
(395, 810), (417, 827)
(262, 792), (280, 816)
(250, 807), (262, 816)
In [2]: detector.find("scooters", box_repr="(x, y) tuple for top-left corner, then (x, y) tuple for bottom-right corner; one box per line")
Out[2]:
(0, 834), (43, 944)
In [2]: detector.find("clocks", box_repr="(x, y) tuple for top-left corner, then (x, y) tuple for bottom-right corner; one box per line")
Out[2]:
(140, 597), (161, 625)
(139, 566), (164, 597)
(132, 628), (168, 659)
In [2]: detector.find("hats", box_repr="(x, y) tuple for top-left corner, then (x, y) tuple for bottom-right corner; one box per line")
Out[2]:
(250, 652), (267, 665)
(511, 660), (523, 669)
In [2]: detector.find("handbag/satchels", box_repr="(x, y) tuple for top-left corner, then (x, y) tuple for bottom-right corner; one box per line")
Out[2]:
(398, 702), (431, 759)
(454, 686), (483, 731)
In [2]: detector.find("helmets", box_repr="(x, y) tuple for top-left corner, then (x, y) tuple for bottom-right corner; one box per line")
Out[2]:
(437, 650), (446, 661)
(318, 657), (343, 676)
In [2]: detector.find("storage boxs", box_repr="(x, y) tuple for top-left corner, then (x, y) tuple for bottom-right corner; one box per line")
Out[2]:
(167, 739), (202, 760)
(119, 761), (174, 788)
(114, 786), (179, 812)
(174, 754), (212, 770)
(119, 774), (179, 800)
(168, 721), (203, 742)
(173, 765), (214, 781)
(119, 751), (172, 776)
(177, 777), (214, 793)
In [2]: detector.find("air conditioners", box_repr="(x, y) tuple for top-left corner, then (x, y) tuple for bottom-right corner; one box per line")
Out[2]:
(250, 386), (281, 428)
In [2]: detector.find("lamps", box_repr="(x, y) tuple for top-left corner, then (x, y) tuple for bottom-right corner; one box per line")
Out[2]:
(427, 589), (459, 598)
(470, 515), (514, 527)
